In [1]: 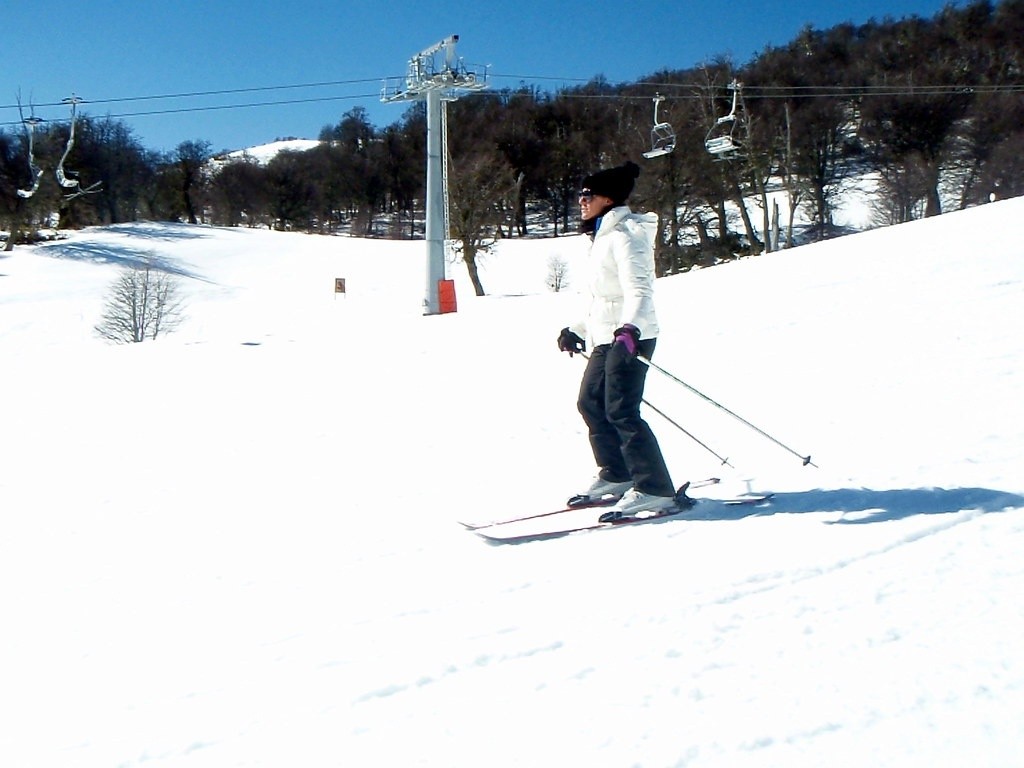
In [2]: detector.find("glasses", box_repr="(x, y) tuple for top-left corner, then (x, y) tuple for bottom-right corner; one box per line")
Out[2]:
(578, 188), (596, 206)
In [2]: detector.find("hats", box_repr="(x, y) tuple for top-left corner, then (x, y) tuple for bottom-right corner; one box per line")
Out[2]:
(583, 159), (642, 206)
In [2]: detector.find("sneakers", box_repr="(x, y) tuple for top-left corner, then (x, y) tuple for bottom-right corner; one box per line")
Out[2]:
(582, 475), (637, 501)
(607, 486), (676, 519)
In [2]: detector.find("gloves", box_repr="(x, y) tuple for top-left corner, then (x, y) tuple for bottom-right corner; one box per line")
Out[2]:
(605, 324), (640, 387)
(558, 328), (585, 356)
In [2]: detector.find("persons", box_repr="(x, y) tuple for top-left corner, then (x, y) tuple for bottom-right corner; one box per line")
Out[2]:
(557, 161), (678, 522)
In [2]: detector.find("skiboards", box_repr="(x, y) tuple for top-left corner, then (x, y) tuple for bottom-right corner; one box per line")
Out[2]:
(455, 477), (775, 540)
